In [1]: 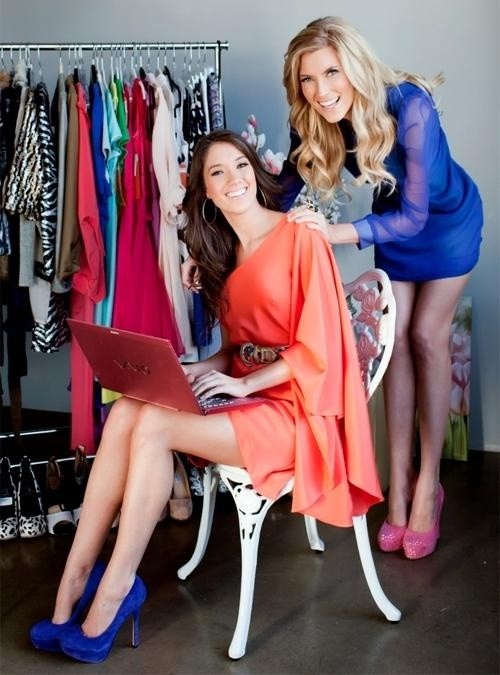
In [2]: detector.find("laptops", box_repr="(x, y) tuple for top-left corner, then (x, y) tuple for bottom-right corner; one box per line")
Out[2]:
(63, 315), (270, 415)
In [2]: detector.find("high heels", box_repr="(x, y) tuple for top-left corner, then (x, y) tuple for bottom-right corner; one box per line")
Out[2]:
(30, 564), (105, 655)
(60, 574), (148, 665)
(377, 470), (419, 553)
(403, 483), (445, 560)
(0, 457), (19, 542)
(71, 444), (89, 532)
(43, 455), (78, 538)
(17, 456), (47, 539)
(168, 451), (193, 521)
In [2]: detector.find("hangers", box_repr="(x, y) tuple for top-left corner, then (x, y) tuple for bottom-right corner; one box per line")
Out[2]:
(0, 42), (207, 102)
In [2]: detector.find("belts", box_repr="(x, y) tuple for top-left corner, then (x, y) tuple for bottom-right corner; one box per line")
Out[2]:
(239, 343), (290, 367)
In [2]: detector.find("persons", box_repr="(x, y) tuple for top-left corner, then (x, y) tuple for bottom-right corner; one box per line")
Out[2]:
(28, 128), (386, 664)
(273, 15), (483, 561)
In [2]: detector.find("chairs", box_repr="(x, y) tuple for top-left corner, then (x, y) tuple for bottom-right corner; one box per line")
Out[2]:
(178, 269), (401, 661)
